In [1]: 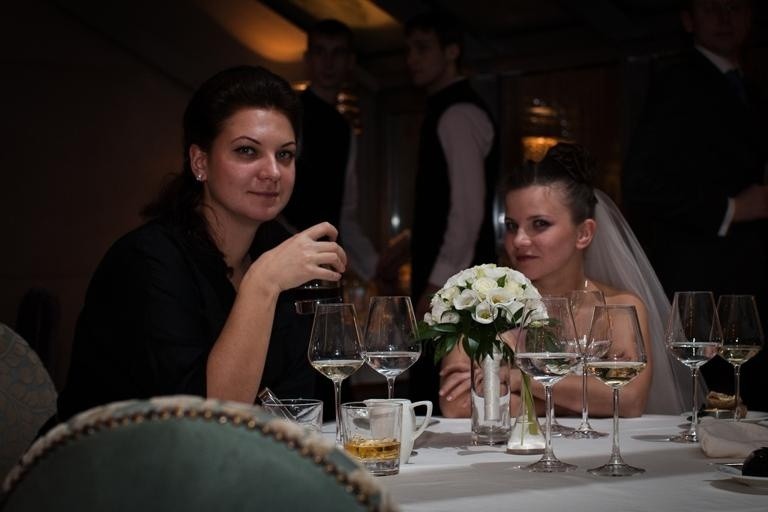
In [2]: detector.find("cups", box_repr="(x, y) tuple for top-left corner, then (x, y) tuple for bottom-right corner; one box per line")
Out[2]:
(294, 262), (344, 316)
(340, 400), (400, 478)
(364, 399), (434, 466)
(262, 399), (323, 441)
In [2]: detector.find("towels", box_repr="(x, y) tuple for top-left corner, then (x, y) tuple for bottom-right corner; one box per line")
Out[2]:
(701, 418), (768, 458)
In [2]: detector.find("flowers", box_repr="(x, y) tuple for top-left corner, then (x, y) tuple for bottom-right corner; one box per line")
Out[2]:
(409, 263), (568, 421)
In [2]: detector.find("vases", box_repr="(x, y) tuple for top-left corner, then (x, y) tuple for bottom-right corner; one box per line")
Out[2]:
(507, 374), (546, 455)
(470, 341), (511, 447)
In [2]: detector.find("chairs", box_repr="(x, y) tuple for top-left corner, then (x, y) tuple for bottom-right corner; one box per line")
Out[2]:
(0, 322), (58, 486)
(0, 394), (398, 511)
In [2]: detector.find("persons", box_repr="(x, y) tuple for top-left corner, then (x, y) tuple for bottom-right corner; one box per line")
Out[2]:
(25, 65), (347, 447)
(436, 154), (653, 421)
(276, 18), (362, 272)
(398, 14), (499, 416)
(614, 1), (768, 411)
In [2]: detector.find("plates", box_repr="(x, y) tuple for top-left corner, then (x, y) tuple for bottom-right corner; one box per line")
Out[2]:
(681, 411), (768, 426)
(721, 462), (768, 491)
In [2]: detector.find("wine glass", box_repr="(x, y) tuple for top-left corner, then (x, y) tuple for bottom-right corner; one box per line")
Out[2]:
(307, 297), (424, 451)
(664, 291), (766, 444)
(515, 291), (650, 479)
